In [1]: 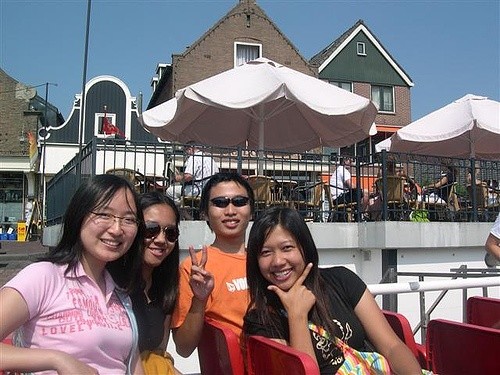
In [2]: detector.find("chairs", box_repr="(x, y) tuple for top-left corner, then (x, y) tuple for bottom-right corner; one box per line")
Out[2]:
(420, 318), (500, 375)
(466, 182), (500, 221)
(180, 175), (216, 219)
(422, 182), (460, 221)
(196, 317), (245, 375)
(245, 335), (321, 375)
(106, 168), (164, 196)
(246, 175), (273, 216)
(452, 191), (473, 222)
(294, 181), (328, 223)
(379, 310), (427, 368)
(326, 183), (359, 221)
(468, 296), (500, 331)
(375, 176), (412, 221)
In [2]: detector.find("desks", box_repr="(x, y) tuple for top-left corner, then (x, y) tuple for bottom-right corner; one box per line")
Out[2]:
(265, 179), (297, 216)
(136, 174), (169, 185)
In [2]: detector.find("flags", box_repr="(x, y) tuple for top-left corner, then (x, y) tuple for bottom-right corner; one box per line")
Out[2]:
(104, 116), (125, 137)
(28, 131), (39, 169)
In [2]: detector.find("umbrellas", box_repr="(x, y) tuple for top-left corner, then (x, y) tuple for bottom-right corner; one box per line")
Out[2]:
(375, 93), (500, 159)
(139, 57), (379, 175)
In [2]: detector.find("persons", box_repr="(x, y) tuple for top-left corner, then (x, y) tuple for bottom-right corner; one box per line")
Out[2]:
(327, 155), (369, 220)
(170, 172), (255, 375)
(165, 142), (221, 221)
(420, 161), (457, 216)
(106, 190), (180, 366)
(485, 212), (500, 262)
(242, 206), (431, 375)
(467, 175), (498, 219)
(371, 157), (421, 217)
(0, 173), (145, 375)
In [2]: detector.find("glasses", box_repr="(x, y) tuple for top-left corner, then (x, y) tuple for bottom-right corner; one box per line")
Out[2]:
(208, 195), (252, 207)
(144, 224), (179, 242)
(91, 212), (140, 227)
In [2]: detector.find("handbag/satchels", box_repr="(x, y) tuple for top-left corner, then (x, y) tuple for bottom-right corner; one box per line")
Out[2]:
(335, 350), (391, 374)
